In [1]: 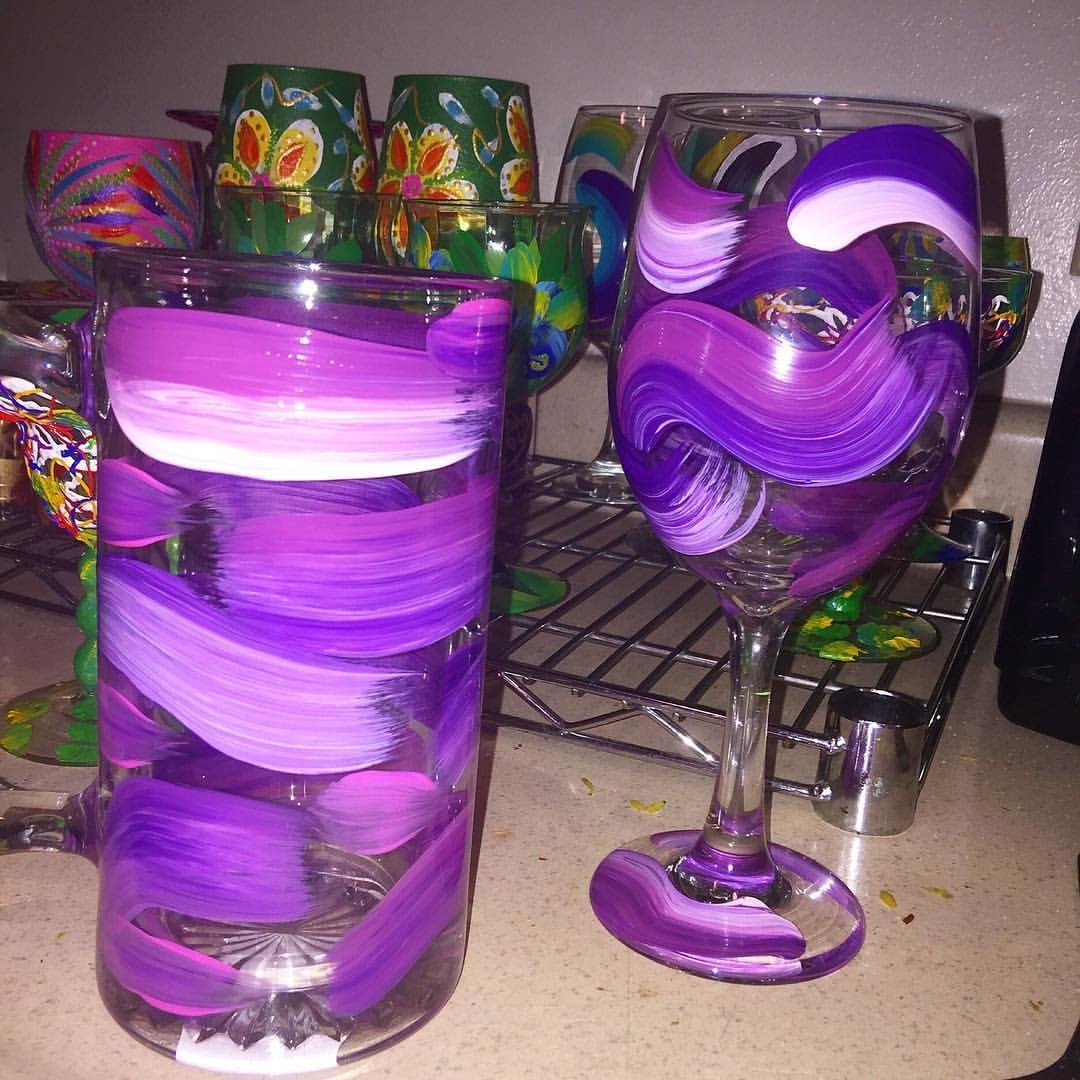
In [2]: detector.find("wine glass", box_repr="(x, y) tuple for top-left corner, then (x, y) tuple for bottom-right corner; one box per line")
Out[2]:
(1, 61), (1041, 987)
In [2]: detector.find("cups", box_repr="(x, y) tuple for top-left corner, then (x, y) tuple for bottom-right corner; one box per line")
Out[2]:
(80, 242), (512, 1079)
